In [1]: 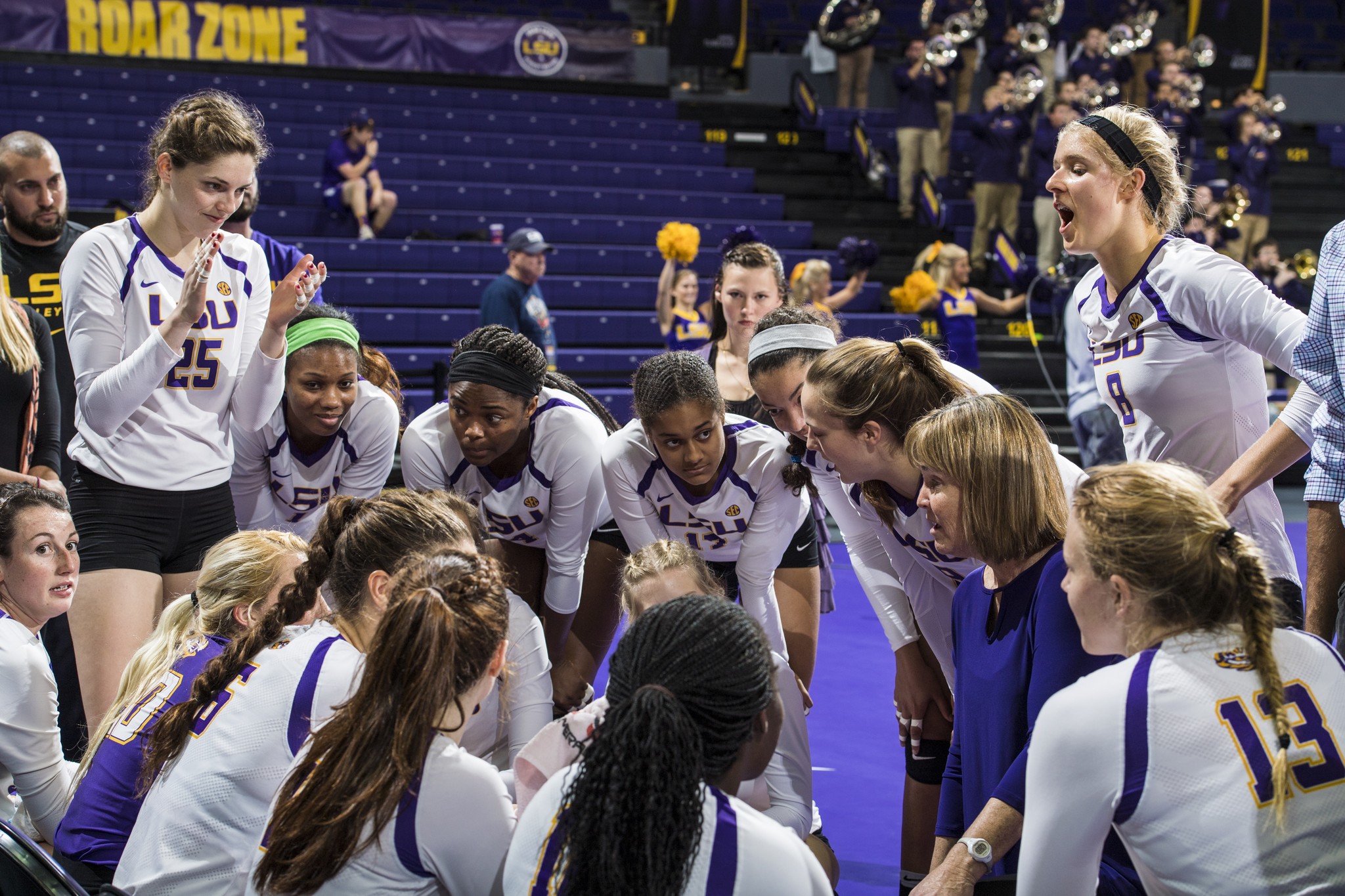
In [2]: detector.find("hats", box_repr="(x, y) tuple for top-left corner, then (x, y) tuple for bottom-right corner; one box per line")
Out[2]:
(506, 227), (556, 255)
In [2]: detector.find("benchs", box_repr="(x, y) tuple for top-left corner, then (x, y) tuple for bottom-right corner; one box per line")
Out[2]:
(0, 54), (1345, 438)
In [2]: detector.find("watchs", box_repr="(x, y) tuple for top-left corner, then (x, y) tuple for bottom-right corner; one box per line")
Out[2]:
(957, 837), (993, 876)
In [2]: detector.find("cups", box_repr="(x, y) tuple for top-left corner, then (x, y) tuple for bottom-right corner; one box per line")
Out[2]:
(490, 224), (504, 245)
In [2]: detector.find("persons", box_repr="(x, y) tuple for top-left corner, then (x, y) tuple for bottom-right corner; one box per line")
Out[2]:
(63, 89), (329, 747)
(0, 0), (1345, 896)
(500, 591), (837, 896)
(1017, 458), (1345, 896)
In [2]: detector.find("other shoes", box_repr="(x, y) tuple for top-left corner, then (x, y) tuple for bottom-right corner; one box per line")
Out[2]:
(359, 224), (375, 240)
(901, 210), (912, 220)
(971, 261), (985, 269)
